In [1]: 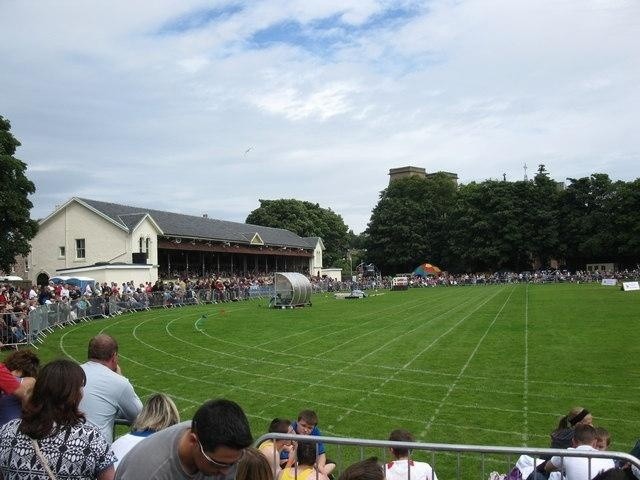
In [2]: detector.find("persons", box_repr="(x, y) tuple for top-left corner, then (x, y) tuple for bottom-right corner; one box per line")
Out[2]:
(544, 405), (594, 461)
(290, 409), (338, 477)
(257, 265), (640, 298)
(0, 264), (185, 353)
(259, 417), (299, 475)
(1, 333), (275, 479)
(276, 442), (332, 480)
(339, 456), (388, 480)
(591, 425), (612, 451)
(185, 268), (255, 305)
(382, 428), (439, 479)
(545, 424), (615, 480)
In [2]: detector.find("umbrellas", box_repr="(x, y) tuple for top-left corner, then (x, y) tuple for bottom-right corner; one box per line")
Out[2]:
(413, 263), (442, 276)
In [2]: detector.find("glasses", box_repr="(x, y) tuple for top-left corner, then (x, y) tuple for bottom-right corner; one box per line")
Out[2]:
(195, 436), (247, 470)
